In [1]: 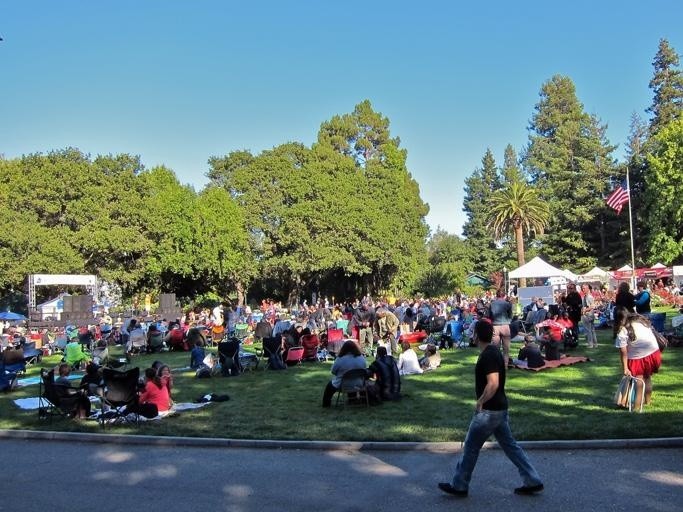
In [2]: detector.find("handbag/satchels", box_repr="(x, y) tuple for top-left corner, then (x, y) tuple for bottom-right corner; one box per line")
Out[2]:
(653, 329), (668, 352)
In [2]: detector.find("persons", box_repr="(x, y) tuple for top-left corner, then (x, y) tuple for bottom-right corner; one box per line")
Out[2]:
(1, 301), (246, 419)
(246, 291), (511, 407)
(511, 281), (682, 411)
(439, 317), (545, 496)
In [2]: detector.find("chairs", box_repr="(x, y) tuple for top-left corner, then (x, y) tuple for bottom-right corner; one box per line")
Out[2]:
(99, 367), (140, 433)
(448, 321), (465, 349)
(39, 369), (88, 426)
(336, 367), (370, 409)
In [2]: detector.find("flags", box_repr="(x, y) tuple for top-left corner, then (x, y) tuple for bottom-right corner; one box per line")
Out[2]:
(606, 174), (629, 214)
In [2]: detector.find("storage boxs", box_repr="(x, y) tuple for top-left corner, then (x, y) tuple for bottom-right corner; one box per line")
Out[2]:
(640, 313), (665, 332)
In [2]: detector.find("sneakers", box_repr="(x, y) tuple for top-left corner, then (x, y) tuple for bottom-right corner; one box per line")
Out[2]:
(439, 483), (467, 495)
(515, 484), (543, 494)
(586, 345), (598, 348)
